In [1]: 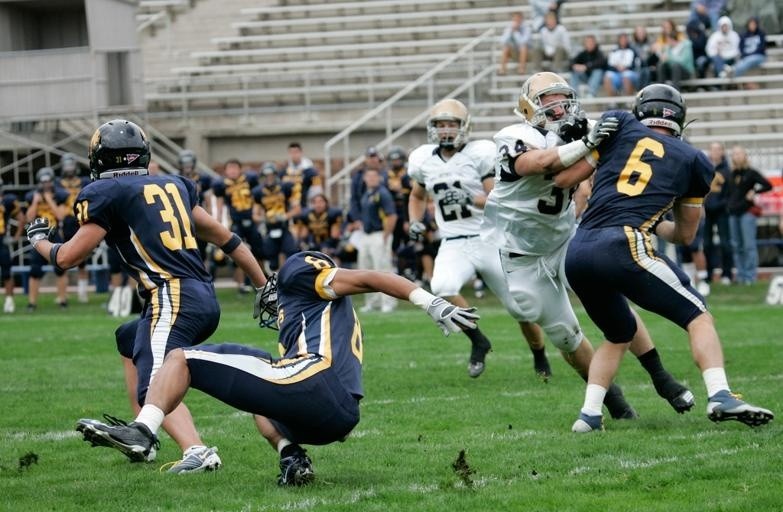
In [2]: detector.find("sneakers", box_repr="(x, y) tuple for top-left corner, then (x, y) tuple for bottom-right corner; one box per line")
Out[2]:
(705, 388), (776, 427)
(355, 299), (398, 316)
(462, 340), (493, 379)
(685, 271), (755, 298)
(603, 381), (638, 425)
(276, 443), (314, 487)
(530, 348), (552, 382)
(569, 411), (606, 436)
(73, 414), (161, 465)
(161, 445), (223, 476)
(0, 285), (142, 321)
(666, 390), (694, 413)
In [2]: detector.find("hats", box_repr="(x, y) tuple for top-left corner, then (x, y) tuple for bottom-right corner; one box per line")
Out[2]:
(363, 144), (380, 159)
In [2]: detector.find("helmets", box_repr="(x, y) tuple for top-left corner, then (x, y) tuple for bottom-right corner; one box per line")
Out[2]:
(424, 96), (470, 153)
(510, 70), (581, 145)
(630, 81), (688, 135)
(36, 149), (279, 182)
(86, 118), (151, 181)
(385, 146), (406, 167)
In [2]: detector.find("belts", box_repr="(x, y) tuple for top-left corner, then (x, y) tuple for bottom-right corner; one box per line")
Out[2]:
(444, 234), (482, 241)
(497, 250), (526, 258)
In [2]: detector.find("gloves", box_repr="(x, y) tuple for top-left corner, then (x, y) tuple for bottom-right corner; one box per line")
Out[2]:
(250, 282), (279, 324)
(24, 218), (51, 242)
(408, 222), (426, 244)
(421, 293), (483, 343)
(585, 115), (620, 151)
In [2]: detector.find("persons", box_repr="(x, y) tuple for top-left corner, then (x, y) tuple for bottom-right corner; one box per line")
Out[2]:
(766, 168), (783, 305)
(76, 251), (479, 486)
(499, 0), (767, 96)
(549, 83), (775, 431)
(406, 99), (552, 380)
(24, 120), (278, 477)
(480, 72), (696, 419)
(1, 143), (772, 316)
